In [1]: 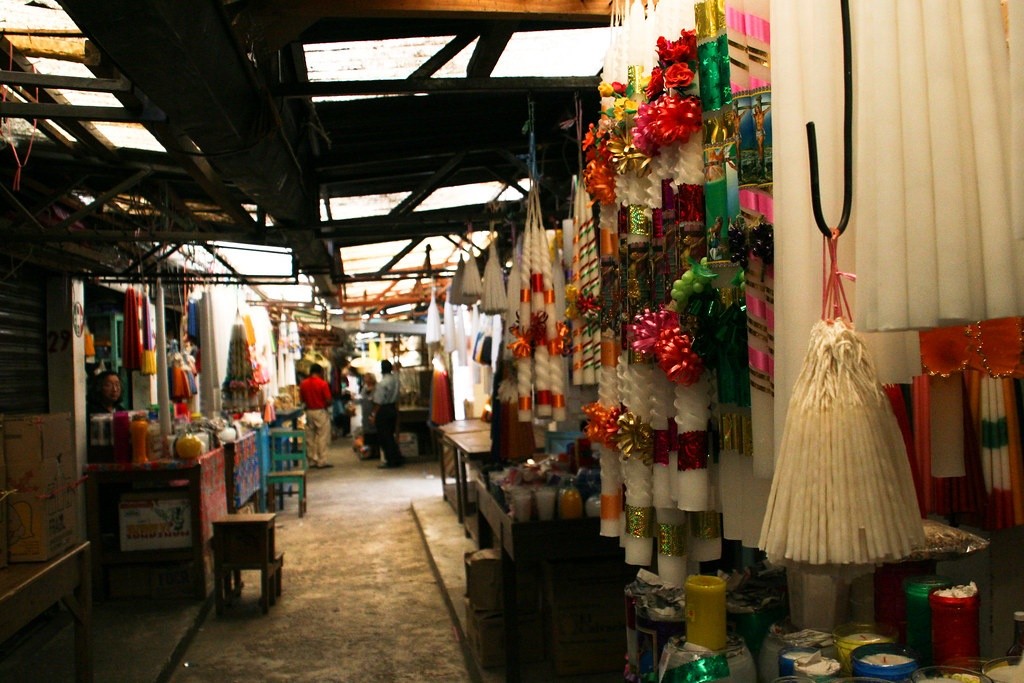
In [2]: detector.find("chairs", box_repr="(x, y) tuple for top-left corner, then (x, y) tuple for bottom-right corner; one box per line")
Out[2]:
(211, 512), (284, 617)
(265, 431), (307, 517)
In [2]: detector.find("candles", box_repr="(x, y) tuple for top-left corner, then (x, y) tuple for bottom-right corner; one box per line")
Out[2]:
(758, 318), (926, 567)
(425, 0), (770, 580)
(684, 575), (727, 649)
(778, 575), (1024, 683)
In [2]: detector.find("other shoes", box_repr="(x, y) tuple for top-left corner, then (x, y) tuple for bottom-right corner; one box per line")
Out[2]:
(309, 464), (317, 468)
(377, 457), (404, 468)
(317, 464), (333, 468)
(342, 433), (353, 437)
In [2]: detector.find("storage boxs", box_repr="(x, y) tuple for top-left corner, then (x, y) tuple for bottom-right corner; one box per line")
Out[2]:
(0, 412), (83, 568)
(118, 497), (193, 551)
(380, 432), (419, 464)
(465, 549), (649, 676)
(110, 561), (195, 598)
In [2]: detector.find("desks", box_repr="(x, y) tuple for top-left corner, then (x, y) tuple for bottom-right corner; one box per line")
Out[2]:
(269, 407), (303, 511)
(476, 465), (658, 683)
(84, 447), (228, 603)
(225, 431), (261, 513)
(396, 404), (436, 455)
(438, 420), (492, 522)
(445, 430), (494, 549)
(0, 541), (93, 683)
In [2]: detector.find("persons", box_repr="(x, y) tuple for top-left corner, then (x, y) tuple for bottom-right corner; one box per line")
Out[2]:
(298, 364), (333, 469)
(331, 366), (377, 461)
(368, 359), (405, 468)
(86, 369), (125, 413)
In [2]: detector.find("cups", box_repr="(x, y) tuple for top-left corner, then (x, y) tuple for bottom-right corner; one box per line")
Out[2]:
(513, 489), (530, 521)
(536, 488), (555, 521)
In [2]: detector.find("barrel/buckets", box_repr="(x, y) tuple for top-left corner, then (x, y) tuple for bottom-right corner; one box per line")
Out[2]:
(902, 573), (954, 668)
(771, 620), (1024, 683)
(663, 632), (756, 683)
(928, 585), (980, 672)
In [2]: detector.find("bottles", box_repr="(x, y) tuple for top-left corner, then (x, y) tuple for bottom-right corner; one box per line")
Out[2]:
(114, 403), (131, 462)
(130, 412), (150, 463)
(146, 411), (164, 461)
(558, 479), (582, 520)
(1007, 611), (1024, 664)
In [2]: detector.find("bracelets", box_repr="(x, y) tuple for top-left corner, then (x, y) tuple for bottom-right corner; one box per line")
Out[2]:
(371, 412), (375, 416)
